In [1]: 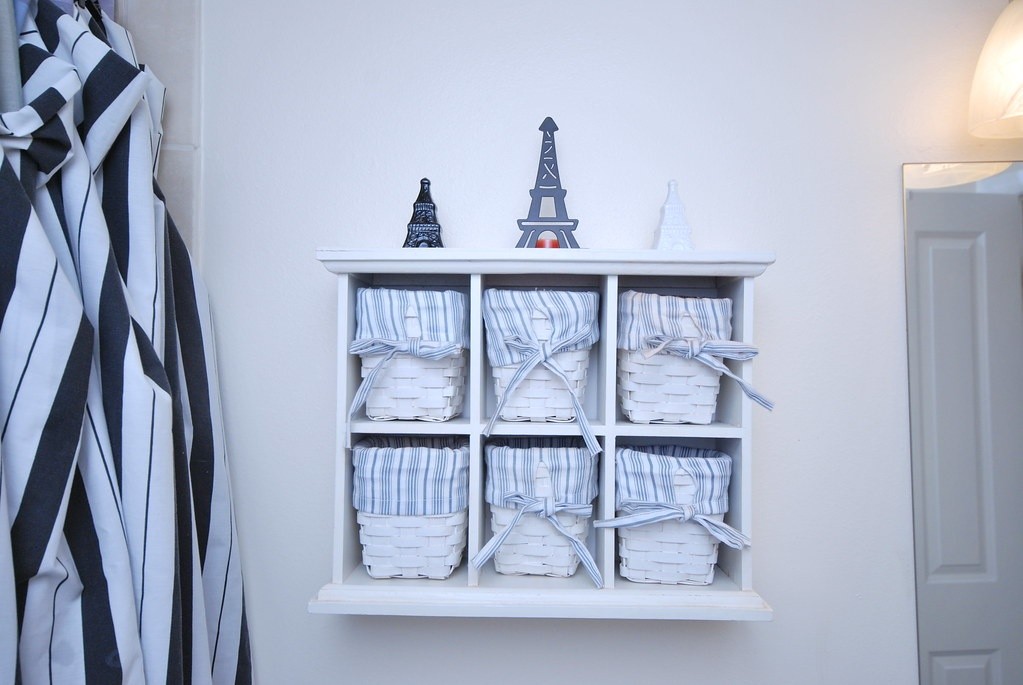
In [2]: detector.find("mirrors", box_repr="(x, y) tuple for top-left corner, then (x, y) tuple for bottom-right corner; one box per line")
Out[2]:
(901, 160), (1023, 685)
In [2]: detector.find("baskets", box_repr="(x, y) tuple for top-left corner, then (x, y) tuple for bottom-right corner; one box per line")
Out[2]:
(352, 434), (469, 581)
(616, 290), (734, 426)
(484, 288), (597, 423)
(615, 445), (732, 584)
(356, 287), (467, 423)
(485, 440), (595, 577)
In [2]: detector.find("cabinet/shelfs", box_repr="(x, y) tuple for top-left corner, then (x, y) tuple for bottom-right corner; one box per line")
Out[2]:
(313, 249), (778, 625)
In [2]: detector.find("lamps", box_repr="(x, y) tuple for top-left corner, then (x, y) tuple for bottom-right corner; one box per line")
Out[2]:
(968, 4), (1023, 139)
